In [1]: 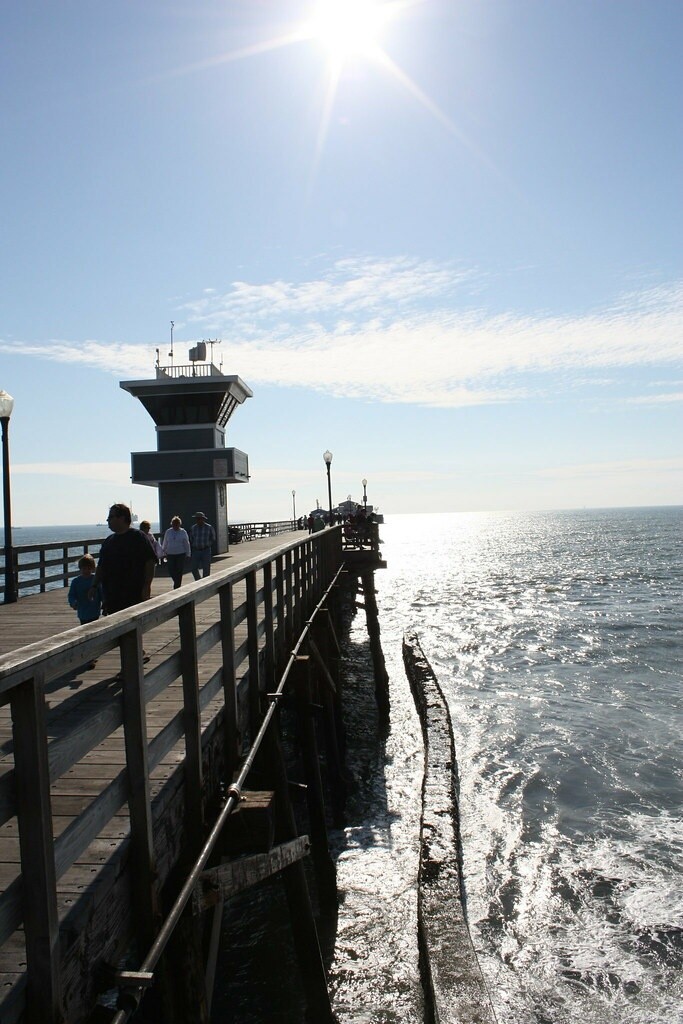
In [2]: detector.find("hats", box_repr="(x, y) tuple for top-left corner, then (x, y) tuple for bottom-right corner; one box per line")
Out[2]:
(191, 512), (209, 520)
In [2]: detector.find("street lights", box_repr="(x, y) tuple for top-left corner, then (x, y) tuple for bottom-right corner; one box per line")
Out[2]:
(292, 490), (295, 526)
(362, 479), (367, 510)
(0, 389), (18, 603)
(323, 450), (333, 527)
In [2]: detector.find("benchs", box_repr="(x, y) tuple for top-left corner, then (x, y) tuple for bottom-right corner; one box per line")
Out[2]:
(245, 529), (256, 541)
(345, 536), (356, 548)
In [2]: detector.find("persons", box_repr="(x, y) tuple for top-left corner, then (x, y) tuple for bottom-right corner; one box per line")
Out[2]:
(137, 521), (165, 597)
(190, 511), (216, 583)
(94, 501), (151, 680)
(161, 516), (192, 591)
(66, 553), (103, 668)
(296, 504), (376, 551)
